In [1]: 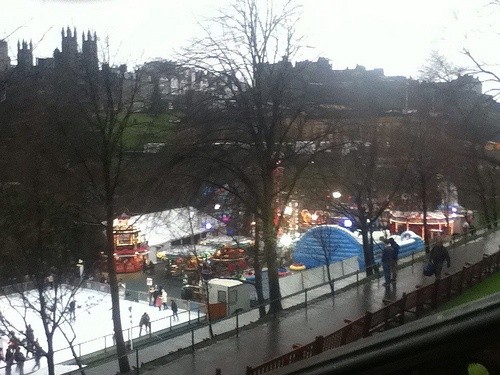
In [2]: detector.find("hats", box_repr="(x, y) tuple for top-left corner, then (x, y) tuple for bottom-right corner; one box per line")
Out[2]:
(436, 238), (443, 243)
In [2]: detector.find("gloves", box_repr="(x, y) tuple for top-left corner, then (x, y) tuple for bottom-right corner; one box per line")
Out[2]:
(447, 264), (450, 268)
(428, 259), (432, 261)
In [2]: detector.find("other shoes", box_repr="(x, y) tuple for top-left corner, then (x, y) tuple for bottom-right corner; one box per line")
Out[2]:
(390, 279), (396, 282)
(383, 282), (389, 285)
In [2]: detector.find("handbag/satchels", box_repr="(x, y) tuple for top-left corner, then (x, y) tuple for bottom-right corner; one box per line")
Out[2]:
(423, 261), (435, 276)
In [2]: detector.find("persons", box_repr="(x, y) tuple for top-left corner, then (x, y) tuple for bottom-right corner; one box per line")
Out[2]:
(70, 300), (76, 320)
(170, 300), (178, 322)
(100, 275), (109, 283)
(143, 259), (154, 274)
(148, 285), (168, 310)
(0, 324), (41, 375)
(140, 312), (150, 335)
(382, 238), (400, 287)
(468, 222), (475, 235)
(429, 240), (450, 280)
